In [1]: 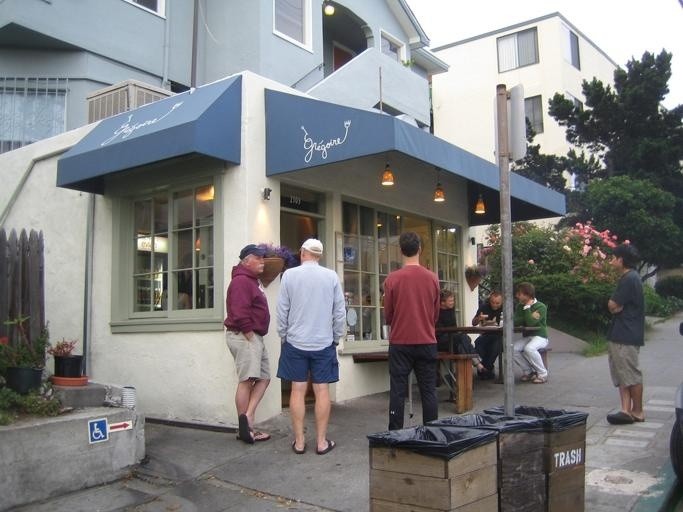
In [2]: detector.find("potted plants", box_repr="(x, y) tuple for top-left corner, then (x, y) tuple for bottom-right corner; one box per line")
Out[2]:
(5, 318), (45, 392)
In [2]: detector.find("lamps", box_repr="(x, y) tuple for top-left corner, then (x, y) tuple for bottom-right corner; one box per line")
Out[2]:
(473, 189), (485, 217)
(382, 154), (399, 189)
(433, 170), (445, 205)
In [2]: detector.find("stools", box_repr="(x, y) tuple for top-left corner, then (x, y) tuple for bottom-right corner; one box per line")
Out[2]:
(493, 342), (553, 383)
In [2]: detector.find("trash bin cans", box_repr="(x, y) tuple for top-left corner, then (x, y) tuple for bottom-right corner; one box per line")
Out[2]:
(366, 406), (590, 512)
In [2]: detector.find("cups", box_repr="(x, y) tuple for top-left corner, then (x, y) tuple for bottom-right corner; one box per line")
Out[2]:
(345, 334), (355, 342)
(382, 325), (389, 339)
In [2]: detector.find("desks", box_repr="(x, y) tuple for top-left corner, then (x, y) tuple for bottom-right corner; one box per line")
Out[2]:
(442, 317), (541, 400)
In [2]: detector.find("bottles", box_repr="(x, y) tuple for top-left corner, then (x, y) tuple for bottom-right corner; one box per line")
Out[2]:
(498, 312), (504, 327)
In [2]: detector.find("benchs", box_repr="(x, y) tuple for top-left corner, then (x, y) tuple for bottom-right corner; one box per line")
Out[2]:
(437, 352), (482, 413)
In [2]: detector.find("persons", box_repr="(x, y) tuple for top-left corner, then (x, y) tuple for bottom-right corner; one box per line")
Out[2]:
(434, 288), (492, 402)
(222, 244), (271, 445)
(274, 237), (346, 454)
(383, 229), (441, 431)
(511, 281), (549, 384)
(471, 289), (503, 379)
(605, 243), (646, 425)
(160, 272), (190, 309)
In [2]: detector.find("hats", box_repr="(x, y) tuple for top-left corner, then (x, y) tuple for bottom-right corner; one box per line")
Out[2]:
(239, 244), (266, 259)
(301, 238), (324, 254)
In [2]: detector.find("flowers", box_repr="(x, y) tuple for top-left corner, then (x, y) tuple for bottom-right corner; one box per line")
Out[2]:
(47, 336), (85, 356)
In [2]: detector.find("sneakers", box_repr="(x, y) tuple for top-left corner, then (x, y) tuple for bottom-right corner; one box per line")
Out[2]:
(520, 373), (548, 384)
(478, 363), (495, 380)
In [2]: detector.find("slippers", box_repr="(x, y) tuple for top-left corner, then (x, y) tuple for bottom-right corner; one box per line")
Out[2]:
(291, 438), (307, 453)
(607, 411), (644, 425)
(316, 439), (336, 454)
(236, 413), (271, 444)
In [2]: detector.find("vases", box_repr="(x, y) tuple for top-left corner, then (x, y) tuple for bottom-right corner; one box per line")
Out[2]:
(52, 356), (83, 380)
